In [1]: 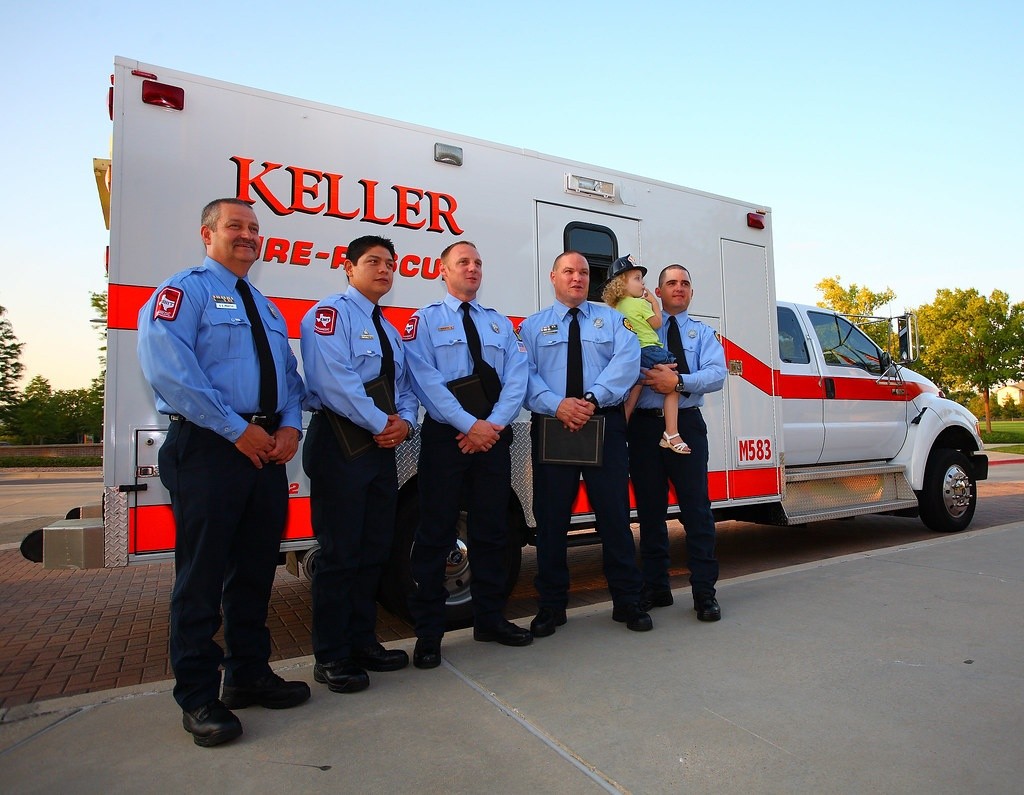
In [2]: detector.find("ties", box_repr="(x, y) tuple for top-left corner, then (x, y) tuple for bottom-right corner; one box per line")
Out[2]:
(370, 305), (396, 403)
(667, 316), (694, 400)
(461, 302), (501, 398)
(565, 308), (583, 400)
(235, 280), (278, 417)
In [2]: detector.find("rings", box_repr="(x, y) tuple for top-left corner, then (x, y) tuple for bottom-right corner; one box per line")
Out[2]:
(391, 439), (394, 444)
(469, 451), (474, 453)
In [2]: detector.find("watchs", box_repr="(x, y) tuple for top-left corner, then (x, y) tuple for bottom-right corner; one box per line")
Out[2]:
(584, 392), (599, 408)
(675, 375), (684, 392)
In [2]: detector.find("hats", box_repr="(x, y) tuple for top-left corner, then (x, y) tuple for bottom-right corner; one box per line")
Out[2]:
(596, 254), (645, 293)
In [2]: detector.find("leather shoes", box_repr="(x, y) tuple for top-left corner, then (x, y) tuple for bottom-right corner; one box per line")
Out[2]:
(218, 668), (312, 710)
(361, 643), (409, 672)
(530, 602), (567, 637)
(612, 601), (651, 631)
(638, 584), (674, 611)
(473, 617), (532, 646)
(693, 590), (721, 622)
(313, 660), (369, 693)
(182, 697), (243, 747)
(414, 632), (445, 669)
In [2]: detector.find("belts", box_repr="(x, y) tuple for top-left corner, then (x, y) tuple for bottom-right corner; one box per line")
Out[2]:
(167, 411), (265, 428)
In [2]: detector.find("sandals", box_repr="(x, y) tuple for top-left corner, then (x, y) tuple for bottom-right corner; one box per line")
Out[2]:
(660, 431), (692, 454)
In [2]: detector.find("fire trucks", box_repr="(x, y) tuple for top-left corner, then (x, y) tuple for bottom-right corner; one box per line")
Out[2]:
(19, 55), (989, 624)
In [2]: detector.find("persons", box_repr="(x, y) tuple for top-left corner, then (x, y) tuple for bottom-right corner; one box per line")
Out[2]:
(520, 250), (653, 638)
(137, 197), (307, 750)
(600, 254), (729, 623)
(403, 242), (533, 668)
(300, 234), (418, 694)
